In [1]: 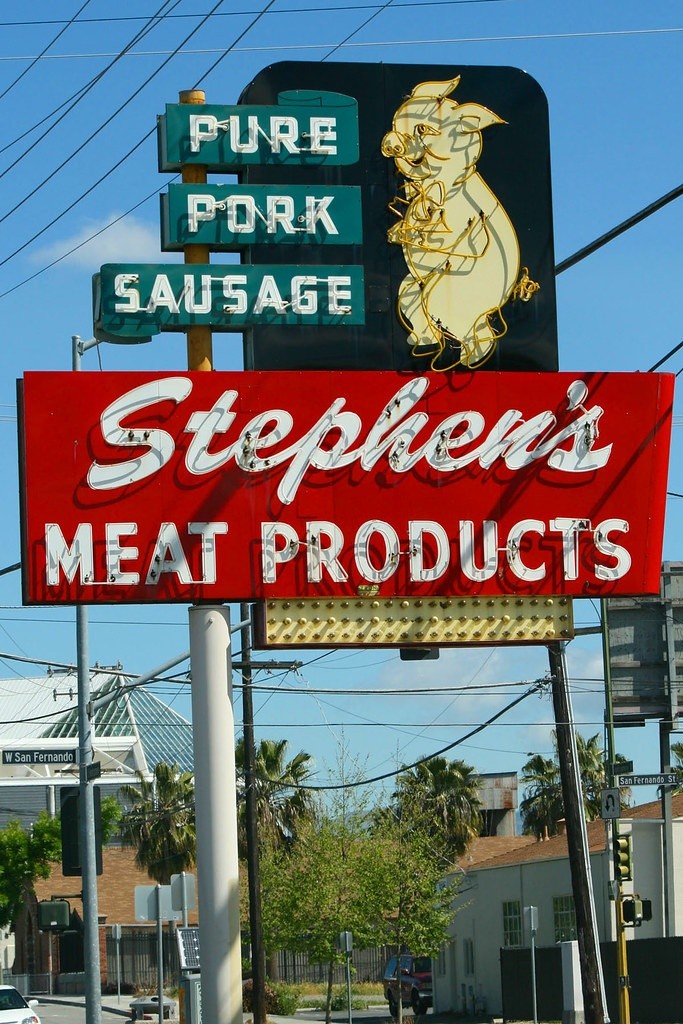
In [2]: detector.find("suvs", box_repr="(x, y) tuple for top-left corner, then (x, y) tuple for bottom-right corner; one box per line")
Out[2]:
(382, 954), (433, 1019)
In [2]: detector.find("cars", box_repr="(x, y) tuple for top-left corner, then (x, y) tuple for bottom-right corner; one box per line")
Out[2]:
(0, 985), (41, 1024)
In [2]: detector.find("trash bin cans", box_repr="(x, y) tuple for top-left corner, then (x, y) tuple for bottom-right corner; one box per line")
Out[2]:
(129, 995), (177, 1024)
